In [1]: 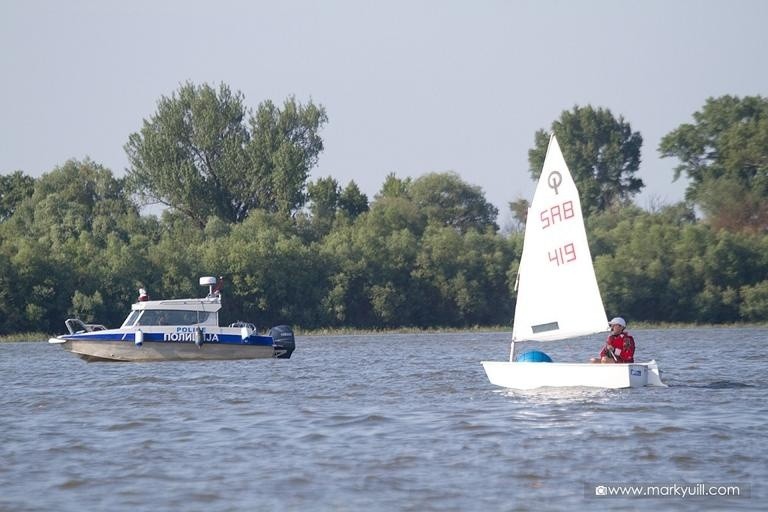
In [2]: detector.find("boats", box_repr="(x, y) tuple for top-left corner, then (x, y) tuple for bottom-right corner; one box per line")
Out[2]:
(47, 276), (303, 363)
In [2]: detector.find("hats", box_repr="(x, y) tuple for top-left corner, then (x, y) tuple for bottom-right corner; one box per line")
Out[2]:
(608, 317), (625, 327)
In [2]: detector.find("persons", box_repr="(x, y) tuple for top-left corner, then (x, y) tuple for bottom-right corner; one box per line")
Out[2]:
(589, 317), (635, 363)
(158, 314), (167, 325)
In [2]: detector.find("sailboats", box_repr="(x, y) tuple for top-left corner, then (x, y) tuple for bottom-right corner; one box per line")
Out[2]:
(480, 128), (667, 393)
